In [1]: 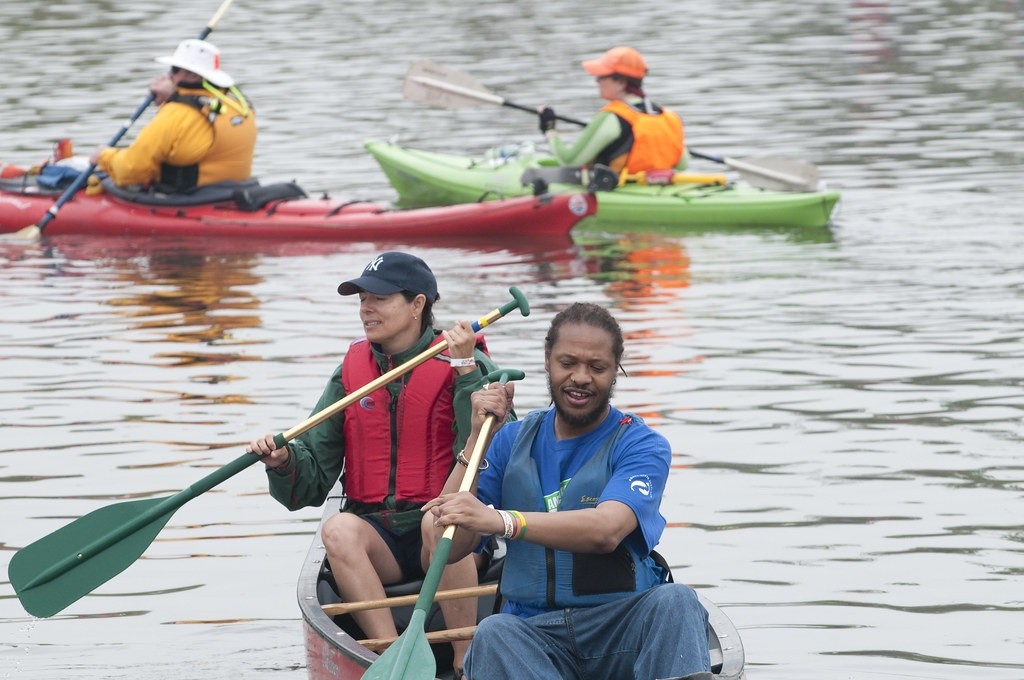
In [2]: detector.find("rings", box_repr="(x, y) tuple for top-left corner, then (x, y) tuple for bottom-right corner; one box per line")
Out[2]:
(483, 384), (488, 390)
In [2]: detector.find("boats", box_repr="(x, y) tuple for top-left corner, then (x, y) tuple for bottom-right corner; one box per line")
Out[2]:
(357, 137), (843, 229)
(0, 138), (617, 244)
(296, 468), (746, 680)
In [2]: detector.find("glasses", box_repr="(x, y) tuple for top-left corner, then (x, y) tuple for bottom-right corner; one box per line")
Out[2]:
(172, 65), (184, 74)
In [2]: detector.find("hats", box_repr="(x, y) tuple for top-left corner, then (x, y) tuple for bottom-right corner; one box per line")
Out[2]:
(337, 251), (437, 305)
(582, 46), (647, 80)
(155, 38), (234, 88)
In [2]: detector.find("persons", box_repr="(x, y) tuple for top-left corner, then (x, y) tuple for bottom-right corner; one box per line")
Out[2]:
(88, 39), (257, 190)
(246, 251), (518, 680)
(421, 302), (712, 680)
(537, 47), (689, 175)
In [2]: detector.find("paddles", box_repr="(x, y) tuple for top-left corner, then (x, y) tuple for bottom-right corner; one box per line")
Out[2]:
(359, 367), (528, 680)
(7, 286), (533, 620)
(9, 0), (234, 241)
(403, 71), (806, 189)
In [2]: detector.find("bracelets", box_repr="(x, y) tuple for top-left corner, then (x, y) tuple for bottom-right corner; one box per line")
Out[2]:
(457, 450), (489, 472)
(497, 510), (513, 539)
(507, 510), (527, 542)
(451, 357), (475, 367)
